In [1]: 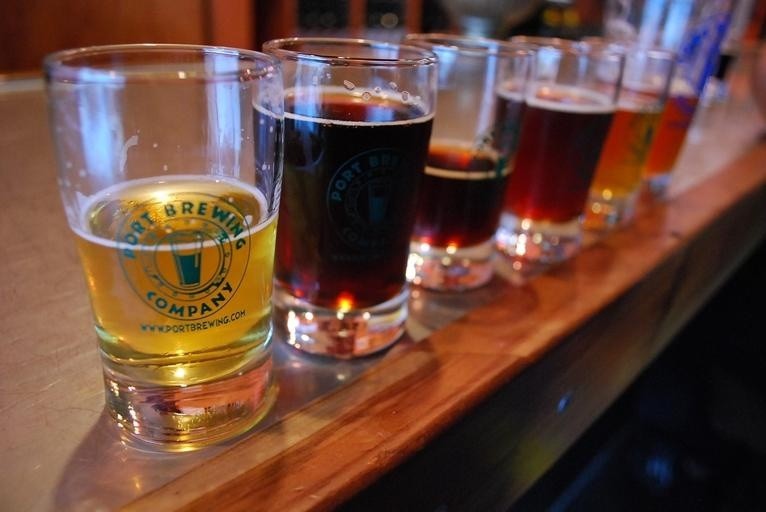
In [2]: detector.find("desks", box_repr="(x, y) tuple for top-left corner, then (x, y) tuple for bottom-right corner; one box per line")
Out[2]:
(1, 61), (765, 510)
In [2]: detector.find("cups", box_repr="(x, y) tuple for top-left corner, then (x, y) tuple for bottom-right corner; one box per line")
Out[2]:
(35, 40), (287, 453)
(260, 32), (435, 362)
(401, 0), (756, 292)
